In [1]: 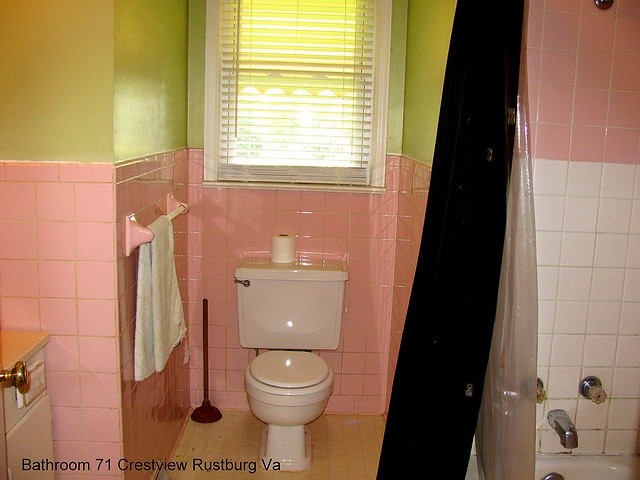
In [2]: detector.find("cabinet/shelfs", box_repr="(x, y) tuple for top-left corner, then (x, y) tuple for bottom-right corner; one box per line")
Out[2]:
(0, 326), (55, 480)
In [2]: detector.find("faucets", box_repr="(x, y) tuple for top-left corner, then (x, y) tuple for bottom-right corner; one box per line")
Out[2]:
(548, 409), (577, 448)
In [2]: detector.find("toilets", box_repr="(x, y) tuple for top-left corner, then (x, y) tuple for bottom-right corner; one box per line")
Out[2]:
(234, 257), (349, 472)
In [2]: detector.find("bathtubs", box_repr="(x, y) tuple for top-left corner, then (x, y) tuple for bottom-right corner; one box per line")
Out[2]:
(464, 454), (639, 479)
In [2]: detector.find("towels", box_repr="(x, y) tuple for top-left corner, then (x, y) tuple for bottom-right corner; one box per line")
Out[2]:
(133, 215), (187, 382)
